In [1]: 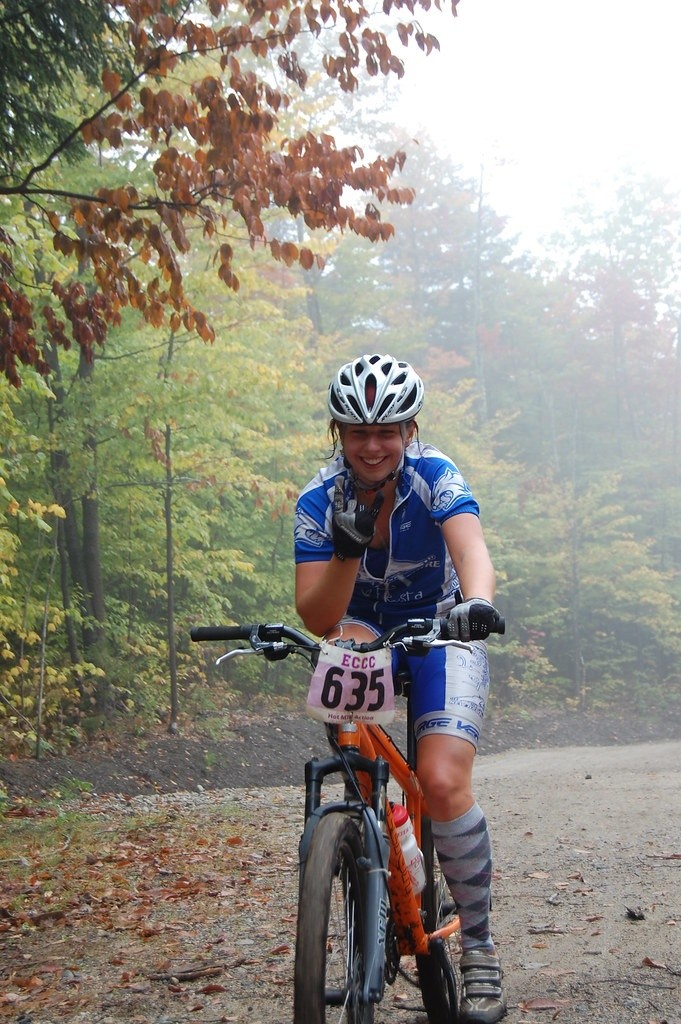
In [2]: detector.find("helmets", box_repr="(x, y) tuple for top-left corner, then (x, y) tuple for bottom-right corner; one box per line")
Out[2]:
(328, 354), (424, 424)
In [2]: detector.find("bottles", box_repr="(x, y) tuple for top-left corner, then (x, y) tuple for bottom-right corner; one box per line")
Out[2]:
(387, 801), (427, 896)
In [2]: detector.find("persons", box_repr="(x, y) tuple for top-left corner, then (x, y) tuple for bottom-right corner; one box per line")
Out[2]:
(294, 354), (506, 1024)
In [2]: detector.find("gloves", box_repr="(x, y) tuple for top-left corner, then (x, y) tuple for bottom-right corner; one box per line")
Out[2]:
(331, 474), (385, 558)
(444, 590), (500, 642)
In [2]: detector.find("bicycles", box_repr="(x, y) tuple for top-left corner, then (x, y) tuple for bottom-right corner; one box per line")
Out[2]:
(189, 617), (507, 1023)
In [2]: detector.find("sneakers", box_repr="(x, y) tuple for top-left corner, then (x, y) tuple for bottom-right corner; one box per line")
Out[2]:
(458, 943), (507, 1024)
